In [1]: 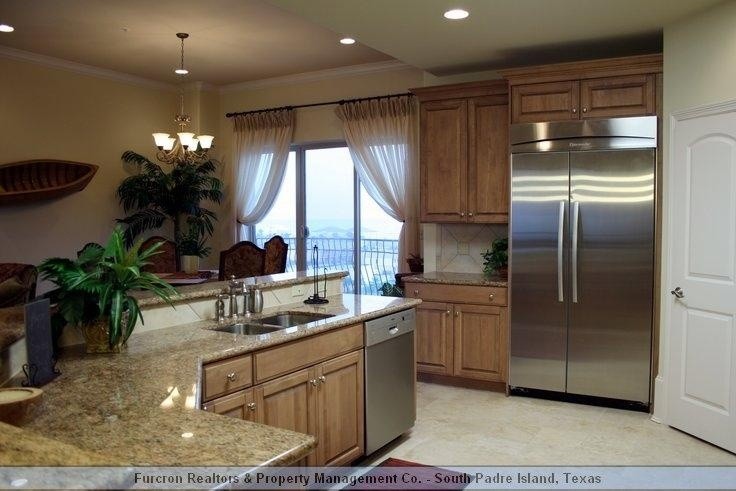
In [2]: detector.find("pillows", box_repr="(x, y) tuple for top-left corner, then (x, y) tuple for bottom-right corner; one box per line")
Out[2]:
(0, 276), (26, 305)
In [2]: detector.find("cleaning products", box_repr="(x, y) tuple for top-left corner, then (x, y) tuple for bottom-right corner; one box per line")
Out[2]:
(248, 276), (264, 313)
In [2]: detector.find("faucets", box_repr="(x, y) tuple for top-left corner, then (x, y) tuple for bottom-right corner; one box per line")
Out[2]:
(222, 275), (248, 318)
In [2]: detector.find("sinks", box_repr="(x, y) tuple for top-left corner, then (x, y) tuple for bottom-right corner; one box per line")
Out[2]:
(250, 310), (335, 328)
(202, 322), (284, 335)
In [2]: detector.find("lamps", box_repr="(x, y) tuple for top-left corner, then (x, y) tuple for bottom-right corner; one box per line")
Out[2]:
(153, 32), (215, 167)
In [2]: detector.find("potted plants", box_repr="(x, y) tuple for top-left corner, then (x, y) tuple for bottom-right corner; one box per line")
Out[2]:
(38, 229), (211, 354)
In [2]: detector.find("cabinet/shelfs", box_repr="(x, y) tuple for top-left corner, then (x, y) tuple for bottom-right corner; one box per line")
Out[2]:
(202, 53), (664, 491)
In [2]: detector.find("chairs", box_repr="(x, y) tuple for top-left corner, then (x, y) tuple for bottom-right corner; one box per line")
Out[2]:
(0, 263), (38, 305)
(77, 235), (288, 292)
